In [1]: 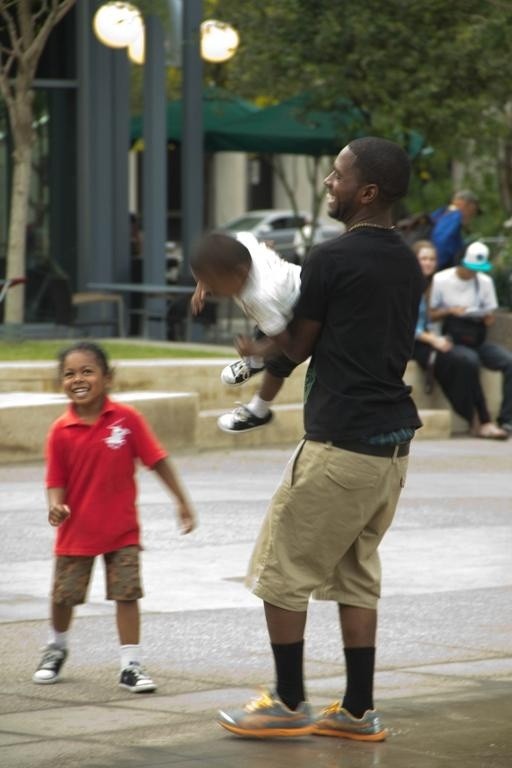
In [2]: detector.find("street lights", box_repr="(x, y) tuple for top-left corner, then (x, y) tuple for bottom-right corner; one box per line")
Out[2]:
(98, 4), (169, 344)
(178, 15), (242, 264)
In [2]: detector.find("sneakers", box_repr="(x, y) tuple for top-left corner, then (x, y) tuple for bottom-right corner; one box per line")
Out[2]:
(220, 360), (266, 386)
(218, 402), (274, 433)
(33, 644), (67, 684)
(217, 685), (389, 742)
(119, 662), (157, 692)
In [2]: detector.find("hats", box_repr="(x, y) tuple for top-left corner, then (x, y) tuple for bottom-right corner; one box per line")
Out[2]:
(453, 188), (486, 215)
(458, 242), (494, 272)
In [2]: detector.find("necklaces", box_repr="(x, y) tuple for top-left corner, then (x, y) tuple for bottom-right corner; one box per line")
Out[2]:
(346, 220), (399, 233)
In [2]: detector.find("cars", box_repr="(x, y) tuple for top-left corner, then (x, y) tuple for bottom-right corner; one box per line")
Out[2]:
(165, 209), (345, 284)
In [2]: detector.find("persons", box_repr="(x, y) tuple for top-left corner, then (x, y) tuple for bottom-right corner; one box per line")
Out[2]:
(212, 134), (433, 744)
(28, 339), (198, 693)
(187, 229), (305, 435)
(410, 183), (512, 443)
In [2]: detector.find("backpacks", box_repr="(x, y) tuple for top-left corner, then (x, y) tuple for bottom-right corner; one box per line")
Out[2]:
(396, 210), (436, 246)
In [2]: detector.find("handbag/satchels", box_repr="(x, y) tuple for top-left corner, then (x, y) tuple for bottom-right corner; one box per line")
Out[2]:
(442, 314), (485, 346)
